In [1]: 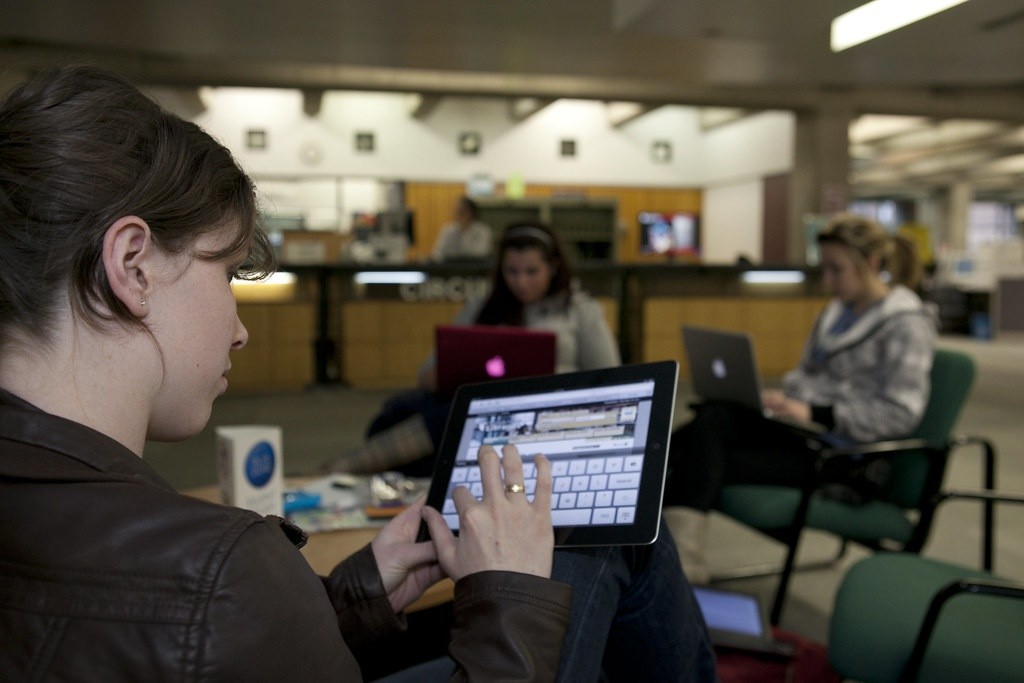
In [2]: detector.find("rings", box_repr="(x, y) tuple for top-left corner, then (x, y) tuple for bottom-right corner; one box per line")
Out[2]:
(505, 483), (525, 494)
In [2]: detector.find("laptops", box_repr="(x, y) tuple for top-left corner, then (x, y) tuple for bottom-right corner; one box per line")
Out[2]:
(433, 326), (556, 403)
(681, 325), (828, 437)
(690, 585), (796, 665)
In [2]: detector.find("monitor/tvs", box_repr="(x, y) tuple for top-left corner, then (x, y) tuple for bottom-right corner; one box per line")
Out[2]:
(634, 207), (702, 261)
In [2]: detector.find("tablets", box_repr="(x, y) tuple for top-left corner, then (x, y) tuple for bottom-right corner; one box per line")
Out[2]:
(416, 360), (680, 550)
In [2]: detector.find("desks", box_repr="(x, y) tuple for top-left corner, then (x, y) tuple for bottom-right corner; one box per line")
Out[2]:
(221, 259), (841, 396)
(178, 476), (457, 616)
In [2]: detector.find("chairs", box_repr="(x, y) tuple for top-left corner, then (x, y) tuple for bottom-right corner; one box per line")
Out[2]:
(686, 346), (1024, 682)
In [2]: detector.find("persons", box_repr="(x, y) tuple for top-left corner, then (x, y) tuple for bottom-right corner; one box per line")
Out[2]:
(429, 194), (495, 265)
(366, 217), (626, 481)
(0, 67), (574, 683)
(665, 215), (938, 517)
